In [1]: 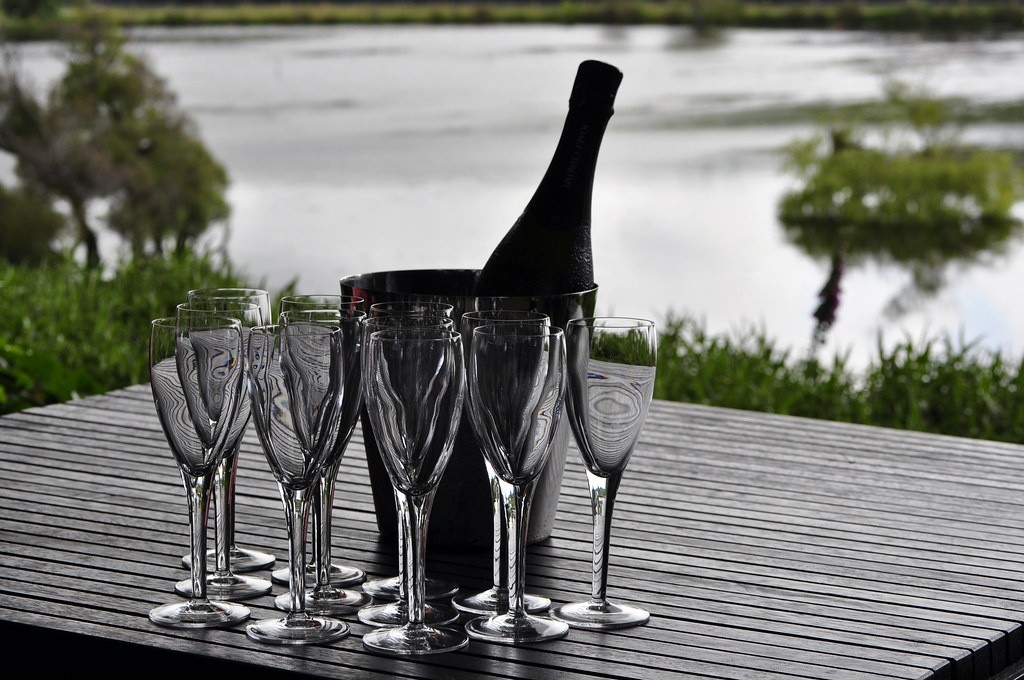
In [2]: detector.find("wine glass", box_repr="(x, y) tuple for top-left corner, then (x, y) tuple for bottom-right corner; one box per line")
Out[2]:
(361, 302), (461, 600)
(450, 306), (553, 613)
(274, 307), (373, 618)
(550, 314), (659, 629)
(171, 303), (263, 601)
(271, 293), (367, 586)
(462, 325), (569, 644)
(183, 286), (279, 570)
(245, 322), (350, 646)
(148, 314), (252, 628)
(362, 330), (464, 656)
(358, 316), (465, 629)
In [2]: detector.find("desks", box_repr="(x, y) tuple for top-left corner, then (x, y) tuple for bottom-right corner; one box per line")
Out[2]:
(0, 380), (1024, 680)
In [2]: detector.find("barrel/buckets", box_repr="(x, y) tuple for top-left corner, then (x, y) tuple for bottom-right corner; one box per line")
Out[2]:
(341, 268), (599, 548)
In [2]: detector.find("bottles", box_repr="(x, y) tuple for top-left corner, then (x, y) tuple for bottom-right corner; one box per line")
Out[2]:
(478, 60), (622, 301)
